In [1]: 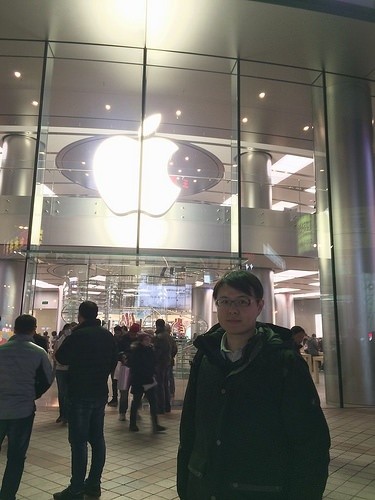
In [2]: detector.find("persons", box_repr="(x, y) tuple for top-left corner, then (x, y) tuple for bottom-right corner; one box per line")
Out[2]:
(290, 325), (305, 344)
(303, 333), (323, 356)
(128, 333), (166, 432)
(55, 322), (78, 422)
(0, 314), (54, 500)
(53, 300), (117, 500)
(96, 317), (178, 421)
(176, 269), (330, 500)
(32, 324), (71, 372)
(0, 324), (16, 344)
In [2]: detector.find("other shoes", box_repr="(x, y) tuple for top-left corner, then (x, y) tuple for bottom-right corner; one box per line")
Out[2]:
(56, 416), (68, 424)
(108, 399), (118, 406)
(155, 425), (167, 431)
(130, 424), (139, 431)
(119, 413), (125, 421)
(84, 481), (101, 498)
(52, 484), (84, 500)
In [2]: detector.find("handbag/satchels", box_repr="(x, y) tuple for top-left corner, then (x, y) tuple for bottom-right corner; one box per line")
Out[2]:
(113, 361), (130, 392)
(142, 376), (157, 391)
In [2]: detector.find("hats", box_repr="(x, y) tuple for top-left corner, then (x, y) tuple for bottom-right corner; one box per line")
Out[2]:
(130, 323), (140, 332)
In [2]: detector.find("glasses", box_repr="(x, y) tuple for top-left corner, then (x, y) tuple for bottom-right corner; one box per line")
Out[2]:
(215, 295), (257, 308)
